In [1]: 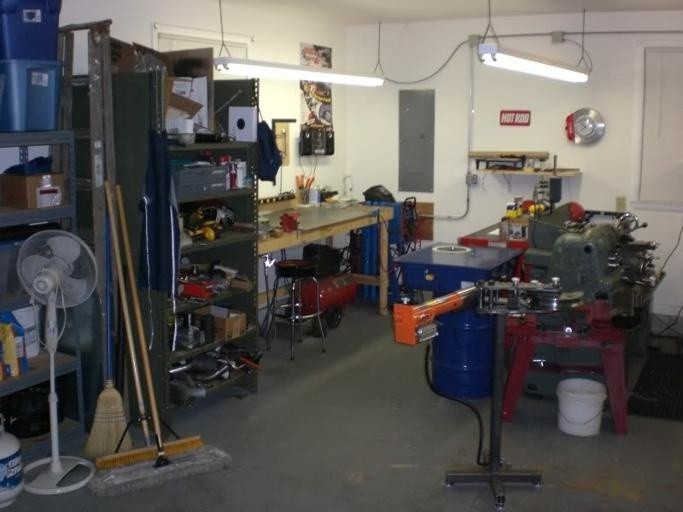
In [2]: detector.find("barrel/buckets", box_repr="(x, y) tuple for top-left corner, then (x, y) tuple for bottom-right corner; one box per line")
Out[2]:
(429, 291), (495, 400)
(556, 377), (607, 439)
(11, 304), (42, 360)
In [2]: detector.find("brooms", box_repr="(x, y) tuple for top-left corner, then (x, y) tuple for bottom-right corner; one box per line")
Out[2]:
(93, 179), (205, 471)
(83, 209), (134, 461)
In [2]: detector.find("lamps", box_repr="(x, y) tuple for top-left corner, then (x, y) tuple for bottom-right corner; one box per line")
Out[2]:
(472, 0), (593, 87)
(204, 1), (388, 92)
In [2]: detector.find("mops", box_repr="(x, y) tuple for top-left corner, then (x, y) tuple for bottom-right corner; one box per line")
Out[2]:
(86, 183), (235, 499)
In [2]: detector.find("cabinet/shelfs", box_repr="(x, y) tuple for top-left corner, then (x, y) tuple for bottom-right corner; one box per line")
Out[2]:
(0, 0), (92, 454)
(64, 66), (266, 435)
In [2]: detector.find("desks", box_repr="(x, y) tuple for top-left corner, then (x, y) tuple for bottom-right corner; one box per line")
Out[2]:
(500, 264), (668, 438)
(454, 217), (533, 282)
(256, 196), (394, 322)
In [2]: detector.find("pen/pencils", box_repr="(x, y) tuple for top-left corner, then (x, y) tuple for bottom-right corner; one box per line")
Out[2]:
(296, 174), (315, 204)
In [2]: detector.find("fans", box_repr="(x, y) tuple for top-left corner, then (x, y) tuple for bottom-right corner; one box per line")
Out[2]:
(12, 225), (104, 498)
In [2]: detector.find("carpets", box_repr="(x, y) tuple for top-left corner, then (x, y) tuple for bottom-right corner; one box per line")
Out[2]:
(622, 343), (683, 424)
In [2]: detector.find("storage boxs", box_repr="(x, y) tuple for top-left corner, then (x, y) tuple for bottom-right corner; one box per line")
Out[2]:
(0, 58), (73, 138)
(1, 0), (65, 58)
(0, 172), (73, 215)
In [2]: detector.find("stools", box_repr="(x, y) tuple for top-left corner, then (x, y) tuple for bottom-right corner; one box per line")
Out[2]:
(264, 257), (327, 362)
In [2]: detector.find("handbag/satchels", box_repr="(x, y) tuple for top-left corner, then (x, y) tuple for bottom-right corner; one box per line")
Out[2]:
(257, 121), (281, 181)
